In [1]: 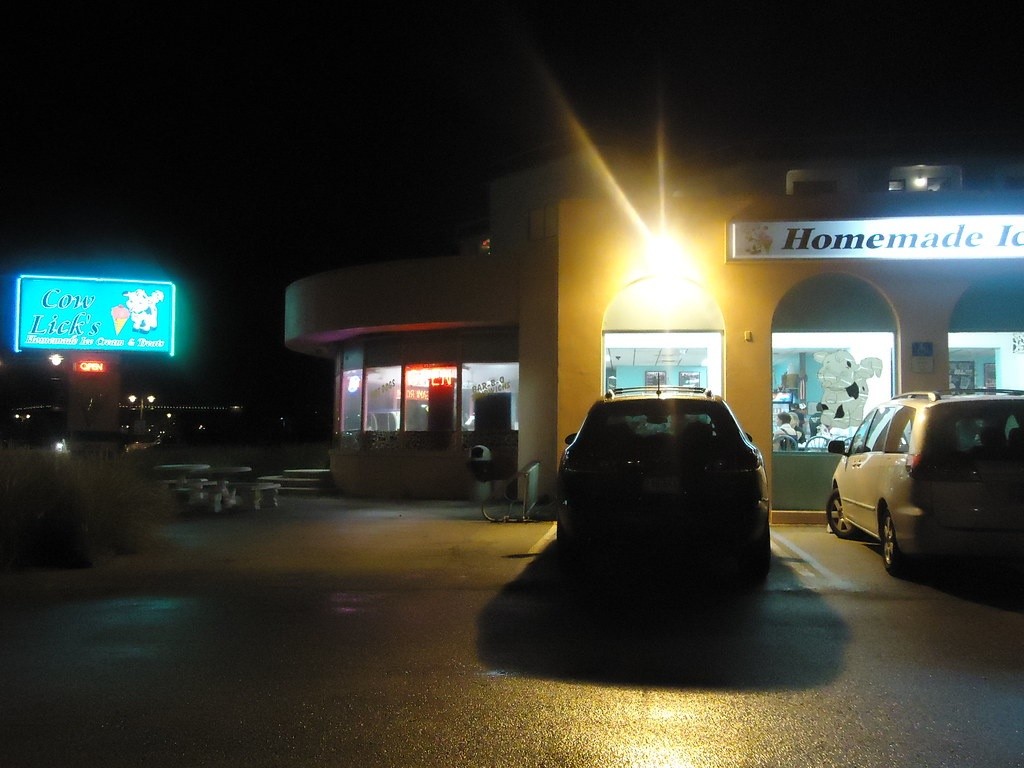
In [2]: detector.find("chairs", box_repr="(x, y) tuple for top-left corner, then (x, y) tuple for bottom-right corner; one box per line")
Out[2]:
(609, 424), (640, 450)
(805, 436), (853, 451)
(971, 428), (1024, 463)
(773, 436), (798, 451)
(677, 422), (713, 446)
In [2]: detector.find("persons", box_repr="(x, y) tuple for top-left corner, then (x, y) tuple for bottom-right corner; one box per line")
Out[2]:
(773, 402), (829, 451)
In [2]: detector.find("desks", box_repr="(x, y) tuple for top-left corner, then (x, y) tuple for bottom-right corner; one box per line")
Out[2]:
(191, 467), (252, 513)
(153, 464), (210, 489)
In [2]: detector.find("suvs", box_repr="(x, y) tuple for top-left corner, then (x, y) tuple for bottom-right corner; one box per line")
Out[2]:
(554, 385), (773, 583)
(825, 388), (1024, 580)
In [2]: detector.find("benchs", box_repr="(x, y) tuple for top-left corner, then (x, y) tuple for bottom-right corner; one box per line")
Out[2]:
(164, 478), (229, 502)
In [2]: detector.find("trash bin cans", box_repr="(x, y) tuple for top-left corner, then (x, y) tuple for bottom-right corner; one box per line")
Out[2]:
(469, 445), (494, 502)
